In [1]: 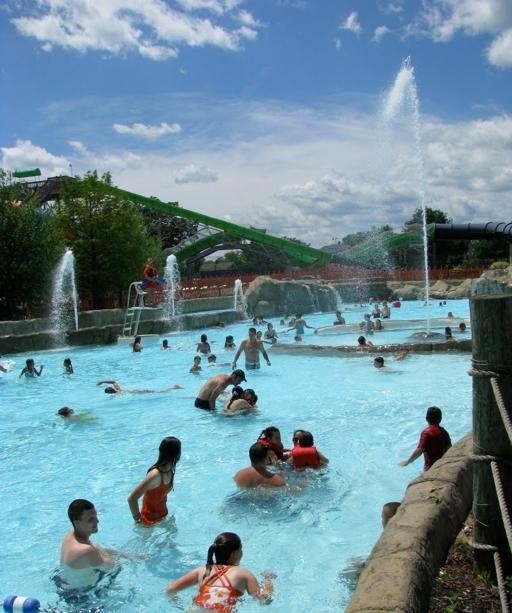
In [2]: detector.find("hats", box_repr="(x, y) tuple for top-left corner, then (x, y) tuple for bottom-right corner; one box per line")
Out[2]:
(234, 369), (247, 382)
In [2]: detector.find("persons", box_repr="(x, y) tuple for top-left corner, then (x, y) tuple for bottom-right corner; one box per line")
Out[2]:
(378, 501), (402, 525)
(55, 499), (146, 598)
(144, 256), (162, 303)
(401, 406), (452, 473)
(0, 295), (468, 423)
(230, 426), (331, 490)
(128, 437), (180, 530)
(166, 532), (275, 610)
